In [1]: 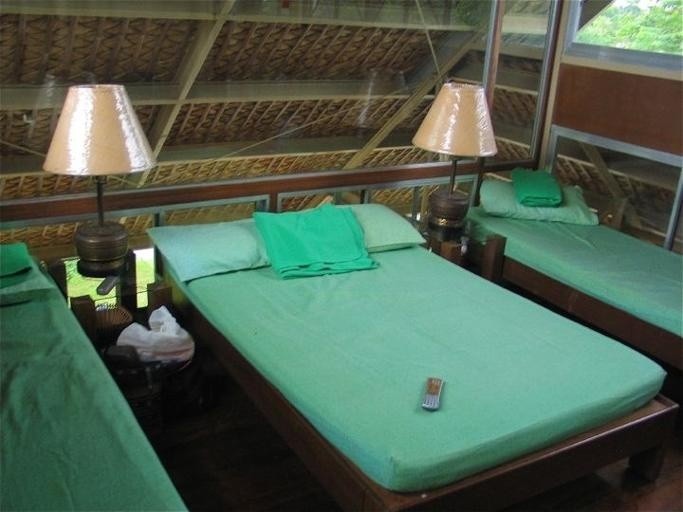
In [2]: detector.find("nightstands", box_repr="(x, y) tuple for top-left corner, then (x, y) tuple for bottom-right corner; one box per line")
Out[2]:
(406, 209), (507, 285)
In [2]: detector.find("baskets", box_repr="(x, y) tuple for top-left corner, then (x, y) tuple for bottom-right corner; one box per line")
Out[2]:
(94, 301), (133, 343)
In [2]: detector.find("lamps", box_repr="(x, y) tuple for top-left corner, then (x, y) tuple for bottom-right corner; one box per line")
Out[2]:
(410, 82), (501, 234)
(45, 84), (156, 278)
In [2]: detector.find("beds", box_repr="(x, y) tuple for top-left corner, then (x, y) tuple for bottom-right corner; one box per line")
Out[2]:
(148, 201), (682, 512)
(467, 202), (680, 374)
(1, 241), (189, 512)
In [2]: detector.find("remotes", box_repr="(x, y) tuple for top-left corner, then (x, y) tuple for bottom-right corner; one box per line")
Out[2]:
(96, 271), (121, 294)
(422, 377), (444, 411)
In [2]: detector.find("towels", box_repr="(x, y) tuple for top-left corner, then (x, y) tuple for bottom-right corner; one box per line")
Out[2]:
(509, 167), (562, 207)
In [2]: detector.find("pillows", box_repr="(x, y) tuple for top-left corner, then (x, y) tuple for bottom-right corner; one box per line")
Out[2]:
(480, 178), (598, 226)
(340, 201), (426, 253)
(145, 222), (275, 280)
(3, 257), (56, 303)
(2, 241), (31, 275)
